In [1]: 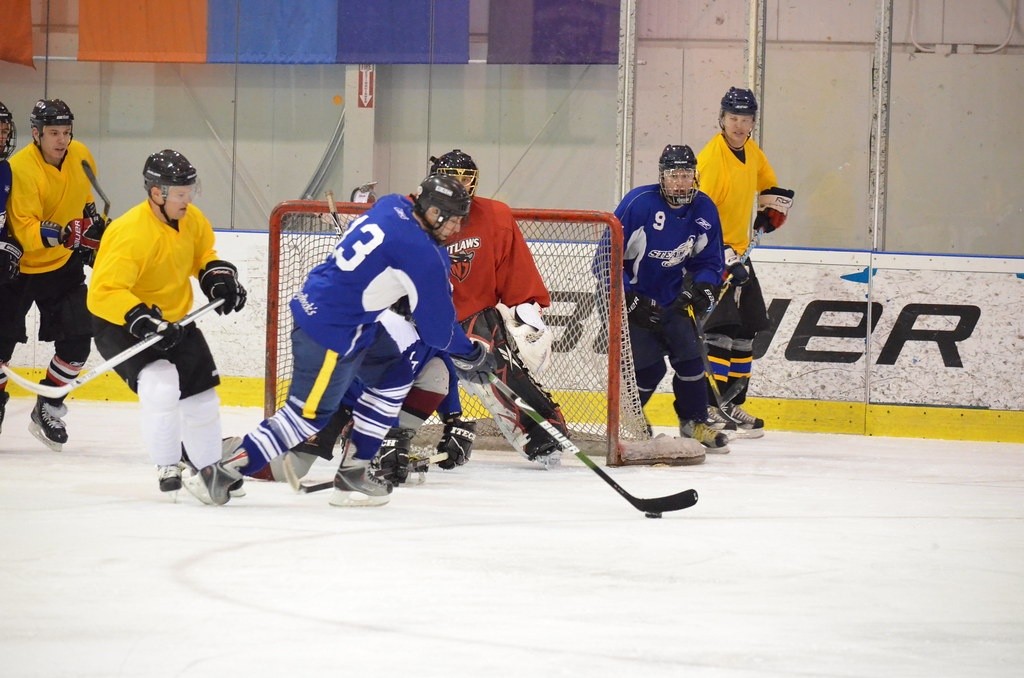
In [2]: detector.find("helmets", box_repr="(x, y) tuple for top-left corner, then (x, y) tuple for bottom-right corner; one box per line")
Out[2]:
(415, 176), (471, 246)
(721, 87), (758, 117)
(430, 151), (478, 202)
(658, 144), (699, 205)
(0, 101), (17, 161)
(29, 100), (74, 136)
(142, 148), (197, 191)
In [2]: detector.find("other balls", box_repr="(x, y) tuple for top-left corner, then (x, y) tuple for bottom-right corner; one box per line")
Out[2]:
(644, 511), (662, 519)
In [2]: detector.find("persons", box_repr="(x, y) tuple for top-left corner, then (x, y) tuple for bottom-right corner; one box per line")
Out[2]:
(181, 296), (476, 487)
(590, 144), (729, 448)
(694, 86), (794, 432)
(0, 98), (107, 443)
(86, 149), (247, 491)
(400, 150), (570, 469)
(201, 173), (496, 510)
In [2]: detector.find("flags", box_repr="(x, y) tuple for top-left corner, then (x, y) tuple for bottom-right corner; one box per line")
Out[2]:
(0, 0), (36, 71)
(76, 0), (620, 65)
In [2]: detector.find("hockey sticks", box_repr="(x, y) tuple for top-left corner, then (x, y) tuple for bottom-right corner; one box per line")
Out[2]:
(686, 298), (747, 408)
(81, 159), (111, 228)
(487, 371), (699, 518)
(283, 451), (449, 493)
(2, 296), (226, 399)
(325, 190), (344, 241)
(698, 224), (764, 327)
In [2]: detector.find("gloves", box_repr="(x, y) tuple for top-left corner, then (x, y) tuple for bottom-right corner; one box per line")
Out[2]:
(0, 234), (24, 282)
(669, 284), (718, 319)
(449, 341), (495, 386)
(123, 304), (187, 352)
(436, 420), (478, 470)
(60, 217), (107, 269)
(719, 245), (752, 286)
(625, 293), (665, 330)
(752, 188), (794, 233)
(197, 260), (247, 316)
(373, 429), (417, 487)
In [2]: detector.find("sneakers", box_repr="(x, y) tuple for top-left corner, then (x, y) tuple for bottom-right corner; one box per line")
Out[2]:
(723, 404), (764, 440)
(158, 463), (184, 504)
(181, 441), (246, 505)
(673, 400), (730, 454)
(181, 436), (249, 506)
(28, 381), (67, 453)
(330, 442), (393, 507)
(0, 390), (9, 431)
(537, 441), (568, 473)
(623, 413), (653, 438)
(705, 406), (740, 442)
